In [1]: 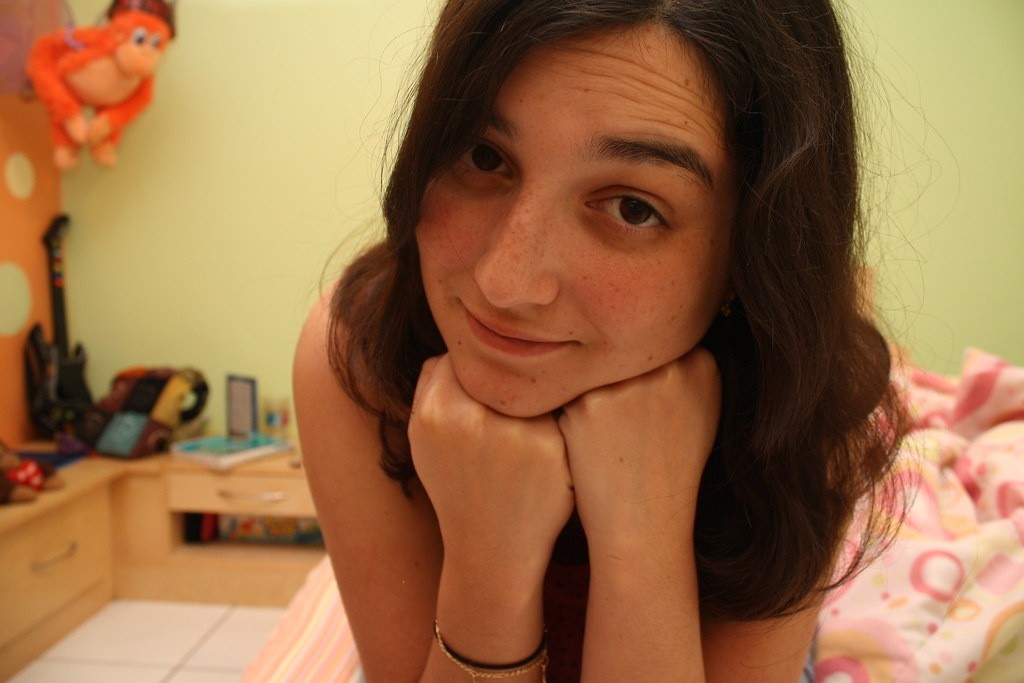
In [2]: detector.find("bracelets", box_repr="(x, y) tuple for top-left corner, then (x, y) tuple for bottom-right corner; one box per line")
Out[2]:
(433, 621), (550, 682)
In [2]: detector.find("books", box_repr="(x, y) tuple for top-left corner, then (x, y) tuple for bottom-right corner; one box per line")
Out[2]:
(170, 434), (287, 468)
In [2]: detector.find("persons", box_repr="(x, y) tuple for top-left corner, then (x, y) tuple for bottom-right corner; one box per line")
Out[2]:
(294, 0), (899, 683)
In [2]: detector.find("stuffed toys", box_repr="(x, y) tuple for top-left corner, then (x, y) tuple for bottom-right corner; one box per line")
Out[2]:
(0, 442), (63, 502)
(27, 0), (175, 168)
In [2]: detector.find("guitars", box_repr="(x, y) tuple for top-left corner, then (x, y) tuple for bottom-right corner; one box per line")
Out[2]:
(18, 211), (96, 442)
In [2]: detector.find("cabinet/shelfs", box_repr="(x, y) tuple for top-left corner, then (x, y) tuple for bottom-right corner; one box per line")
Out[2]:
(0, 434), (348, 683)
